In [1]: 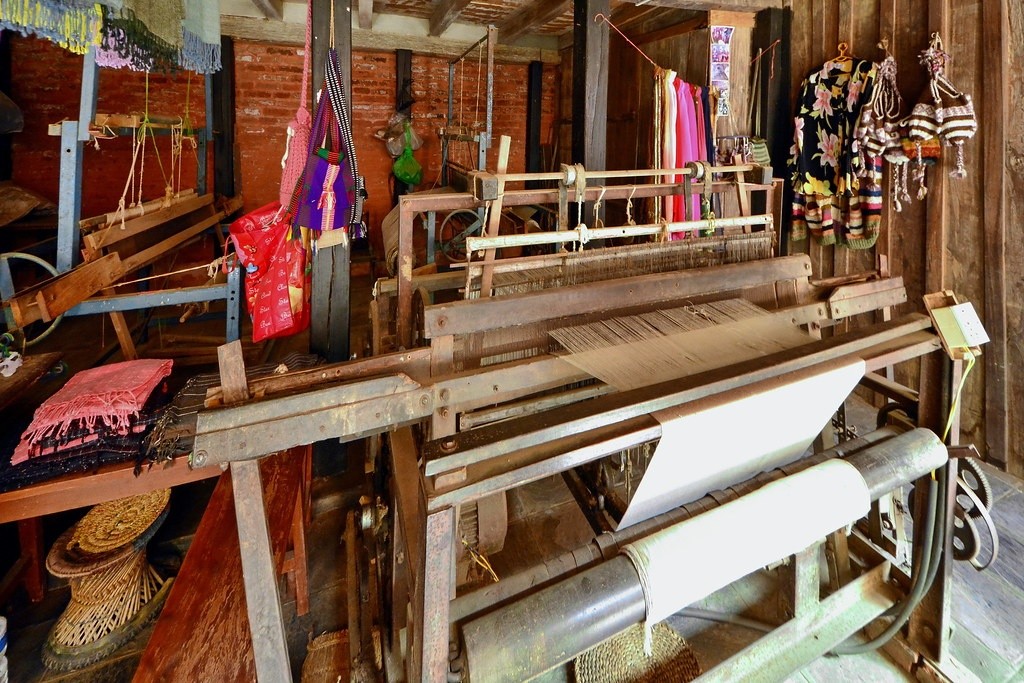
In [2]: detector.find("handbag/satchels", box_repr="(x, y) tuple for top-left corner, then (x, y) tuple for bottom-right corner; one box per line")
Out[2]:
(228, 3), (365, 343)
(856, 71), (981, 214)
(392, 125), (422, 186)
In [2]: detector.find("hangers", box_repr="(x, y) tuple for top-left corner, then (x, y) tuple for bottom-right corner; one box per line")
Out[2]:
(802, 42), (882, 82)
